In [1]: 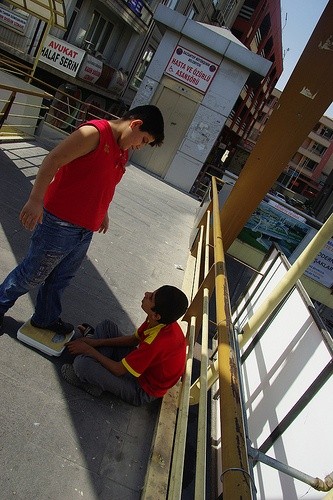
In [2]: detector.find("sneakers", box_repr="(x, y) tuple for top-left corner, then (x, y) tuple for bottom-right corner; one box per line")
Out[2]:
(30, 314), (74, 335)
(0, 314), (4, 336)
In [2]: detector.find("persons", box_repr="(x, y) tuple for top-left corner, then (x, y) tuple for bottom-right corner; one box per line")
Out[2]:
(0, 105), (165, 335)
(62, 285), (188, 407)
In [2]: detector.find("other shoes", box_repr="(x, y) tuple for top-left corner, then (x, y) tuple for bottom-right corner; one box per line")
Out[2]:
(60, 362), (104, 398)
(77, 322), (95, 336)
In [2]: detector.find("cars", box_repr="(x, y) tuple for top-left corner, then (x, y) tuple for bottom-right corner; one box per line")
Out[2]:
(269, 188), (306, 213)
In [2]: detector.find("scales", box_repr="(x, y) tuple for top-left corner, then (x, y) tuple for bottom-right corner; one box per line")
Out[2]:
(16, 312), (76, 357)
